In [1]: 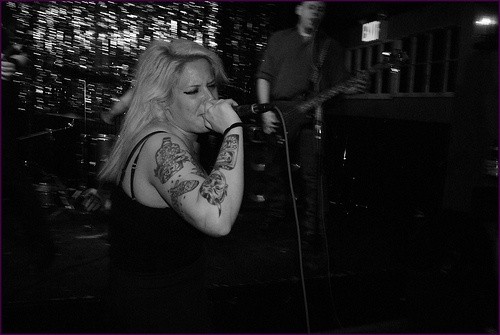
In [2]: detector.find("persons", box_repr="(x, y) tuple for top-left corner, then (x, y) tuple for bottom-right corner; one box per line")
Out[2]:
(95, 37), (246, 335)
(256, 0), (371, 269)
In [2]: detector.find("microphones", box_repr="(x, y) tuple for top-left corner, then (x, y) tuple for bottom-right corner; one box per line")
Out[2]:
(231, 102), (273, 117)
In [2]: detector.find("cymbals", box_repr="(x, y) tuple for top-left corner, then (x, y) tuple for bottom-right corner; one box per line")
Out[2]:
(45, 112), (96, 121)
(44, 63), (117, 84)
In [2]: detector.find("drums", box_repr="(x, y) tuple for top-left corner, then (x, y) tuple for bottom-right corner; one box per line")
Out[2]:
(79, 133), (119, 176)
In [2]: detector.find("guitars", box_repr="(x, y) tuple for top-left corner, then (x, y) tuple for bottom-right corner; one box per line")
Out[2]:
(261, 49), (409, 150)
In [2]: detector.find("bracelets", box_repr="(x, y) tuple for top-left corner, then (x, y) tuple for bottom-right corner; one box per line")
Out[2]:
(260, 102), (271, 114)
(223, 123), (244, 135)
